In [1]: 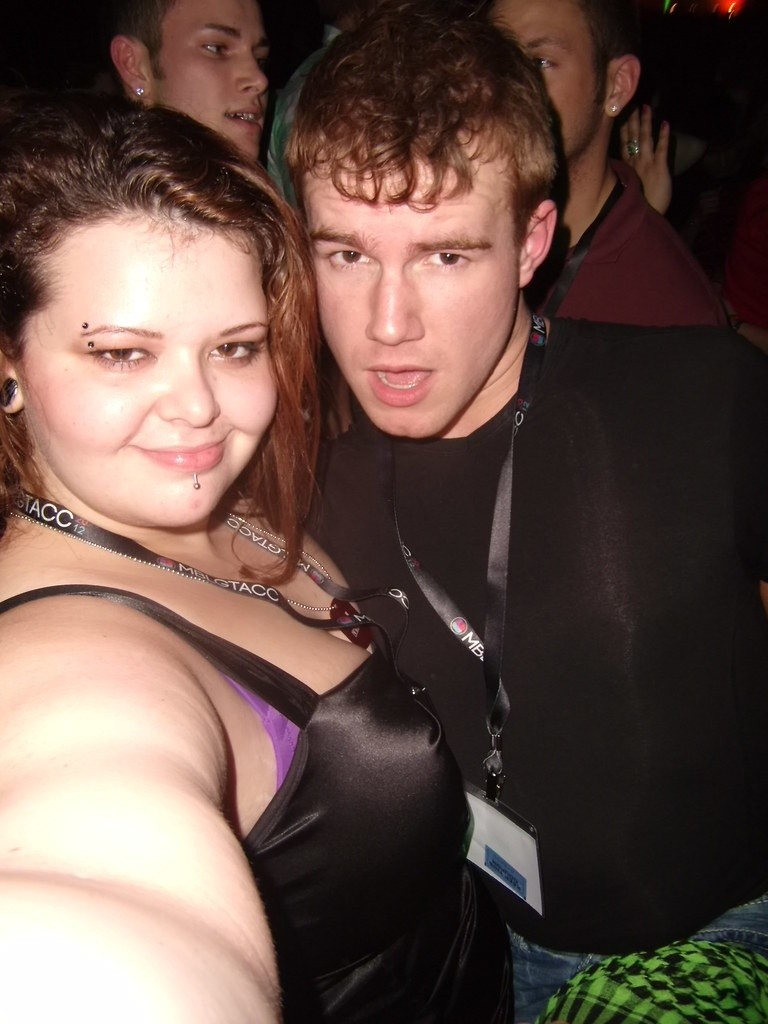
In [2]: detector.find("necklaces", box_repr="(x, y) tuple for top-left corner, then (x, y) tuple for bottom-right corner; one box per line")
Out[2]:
(9, 509), (372, 648)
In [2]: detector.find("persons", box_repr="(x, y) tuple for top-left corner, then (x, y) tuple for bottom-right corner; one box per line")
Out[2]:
(0, 95), (512, 1024)
(286, 0), (768, 1024)
(93, 0), (768, 344)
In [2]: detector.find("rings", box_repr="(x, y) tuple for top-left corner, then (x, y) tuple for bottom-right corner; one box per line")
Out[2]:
(626, 140), (639, 156)
